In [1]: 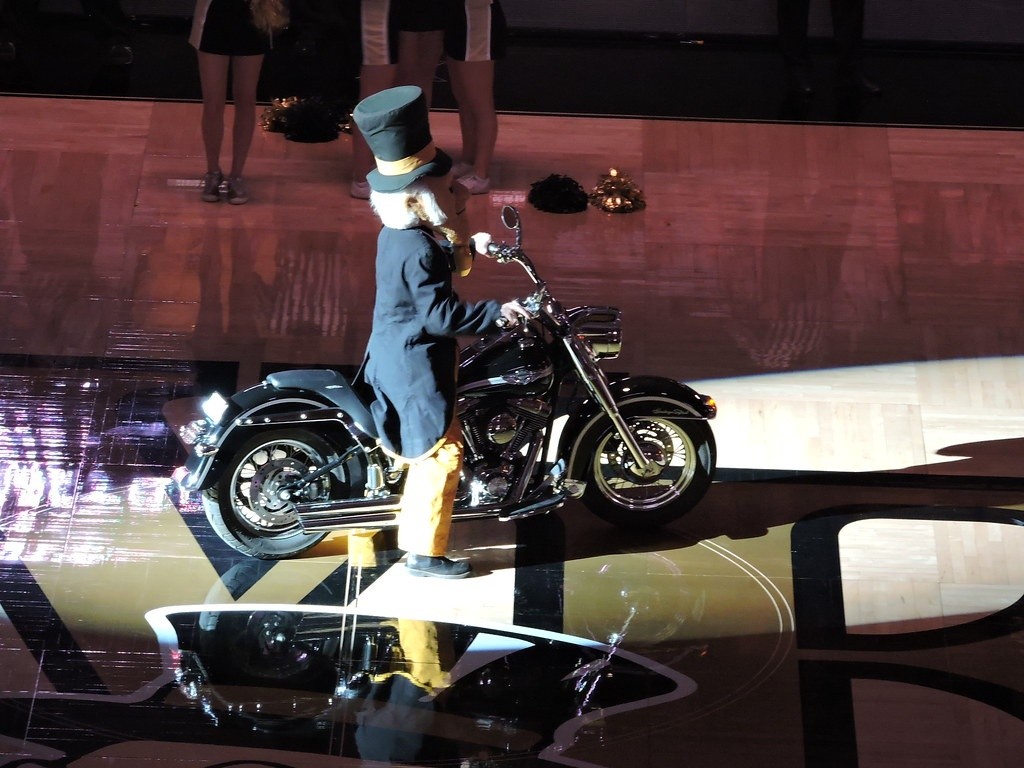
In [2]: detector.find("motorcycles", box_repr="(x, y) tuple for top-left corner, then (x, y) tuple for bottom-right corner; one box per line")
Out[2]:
(179, 201), (717, 564)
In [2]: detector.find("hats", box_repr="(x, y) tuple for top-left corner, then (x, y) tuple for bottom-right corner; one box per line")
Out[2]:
(354, 86), (453, 192)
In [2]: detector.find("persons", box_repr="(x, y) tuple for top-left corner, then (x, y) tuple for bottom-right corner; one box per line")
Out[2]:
(188, 0), (282, 205)
(775, 0), (866, 94)
(350, 0), (507, 200)
(349, 84), (534, 578)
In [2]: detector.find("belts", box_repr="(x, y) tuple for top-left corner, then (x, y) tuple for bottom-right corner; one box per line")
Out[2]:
(406, 554), (472, 578)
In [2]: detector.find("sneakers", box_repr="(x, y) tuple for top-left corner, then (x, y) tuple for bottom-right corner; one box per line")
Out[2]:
(228, 174), (248, 204)
(201, 168), (224, 201)
(457, 172), (491, 194)
(453, 163), (473, 176)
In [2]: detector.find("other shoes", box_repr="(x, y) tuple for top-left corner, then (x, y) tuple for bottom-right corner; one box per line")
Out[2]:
(352, 179), (370, 200)
